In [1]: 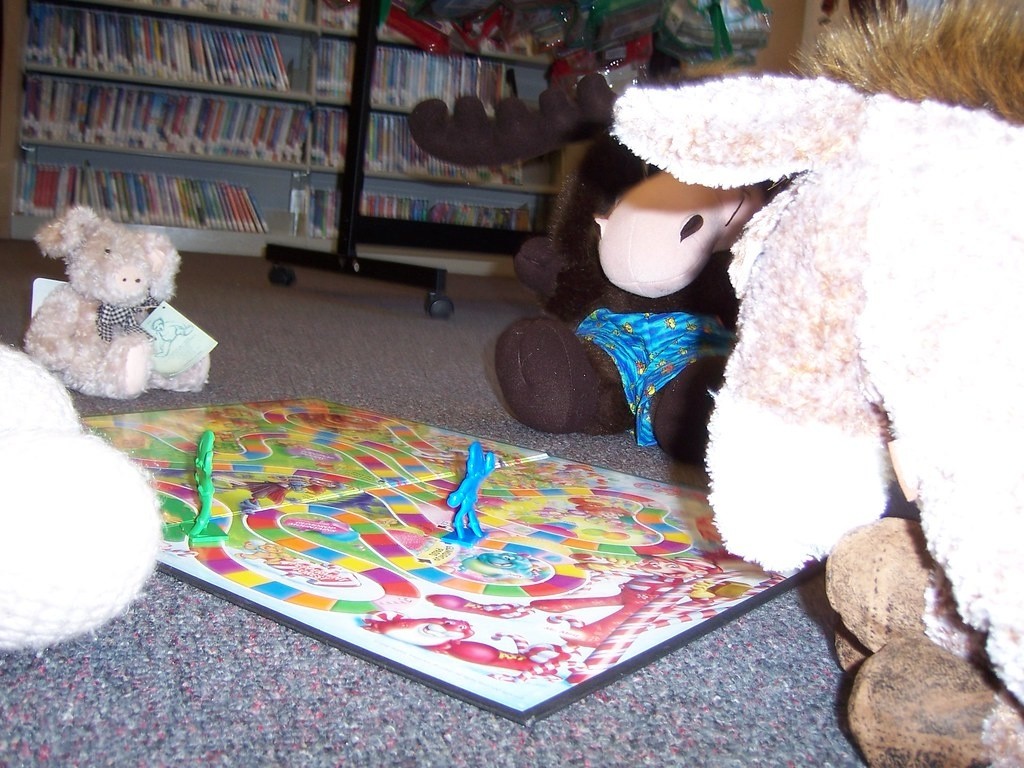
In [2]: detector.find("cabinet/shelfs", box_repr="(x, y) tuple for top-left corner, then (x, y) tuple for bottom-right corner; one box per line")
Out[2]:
(10, 0), (567, 279)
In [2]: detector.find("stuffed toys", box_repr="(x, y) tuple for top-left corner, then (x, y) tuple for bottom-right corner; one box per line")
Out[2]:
(411, 0), (1024, 768)
(22, 206), (210, 399)
(0, 344), (164, 653)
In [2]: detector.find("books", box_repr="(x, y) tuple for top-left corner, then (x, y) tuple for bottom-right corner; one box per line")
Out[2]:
(13, 0), (533, 236)
